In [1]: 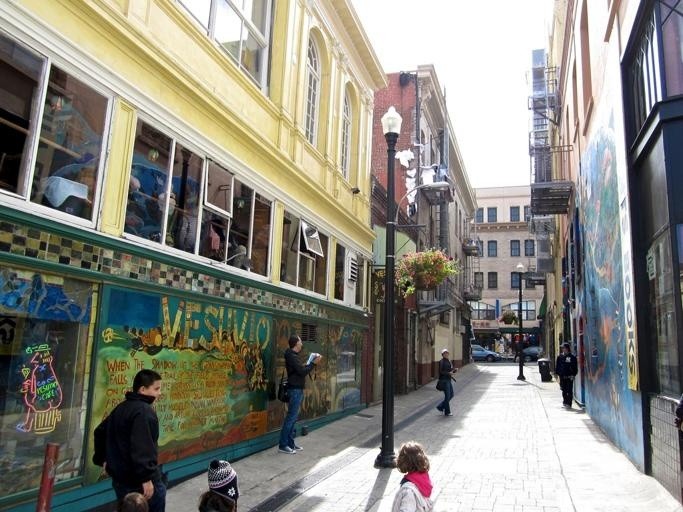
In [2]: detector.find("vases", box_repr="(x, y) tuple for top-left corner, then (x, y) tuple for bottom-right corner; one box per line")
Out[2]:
(415, 283), (435, 291)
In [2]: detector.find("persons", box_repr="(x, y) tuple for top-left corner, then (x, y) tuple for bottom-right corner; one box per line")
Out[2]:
(196, 457), (240, 511)
(118, 492), (149, 511)
(276, 334), (323, 455)
(91, 369), (167, 511)
(555, 342), (578, 408)
(435, 348), (455, 416)
(390, 440), (434, 512)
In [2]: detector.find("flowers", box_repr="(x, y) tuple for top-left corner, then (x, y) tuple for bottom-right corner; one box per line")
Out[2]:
(394, 248), (460, 297)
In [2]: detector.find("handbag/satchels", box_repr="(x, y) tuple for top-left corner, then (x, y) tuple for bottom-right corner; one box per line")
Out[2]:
(436, 381), (446, 391)
(277, 381), (289, 403)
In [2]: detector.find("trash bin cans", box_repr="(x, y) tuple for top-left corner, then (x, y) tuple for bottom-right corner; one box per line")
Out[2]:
(537, 357), (551, 373)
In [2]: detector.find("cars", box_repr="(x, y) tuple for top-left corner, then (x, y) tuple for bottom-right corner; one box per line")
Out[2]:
(515, 346), (544, 362)
(470, 344), (501, 362)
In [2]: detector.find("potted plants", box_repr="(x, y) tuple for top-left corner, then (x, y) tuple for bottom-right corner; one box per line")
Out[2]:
(499, 310), (519, 326)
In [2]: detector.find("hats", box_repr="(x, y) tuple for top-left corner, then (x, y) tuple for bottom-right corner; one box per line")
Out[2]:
(208, 459), (239, 500)
(442, 349), (448, 353)
(559, 343), (569, 348)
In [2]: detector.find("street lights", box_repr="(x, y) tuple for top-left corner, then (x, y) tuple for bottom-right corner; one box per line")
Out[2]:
(371, 102), (449, 470)
(516, 261), (525, 380)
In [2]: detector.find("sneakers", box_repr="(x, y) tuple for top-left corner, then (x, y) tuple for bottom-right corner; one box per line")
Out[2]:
(278, 446), (303, 454)
